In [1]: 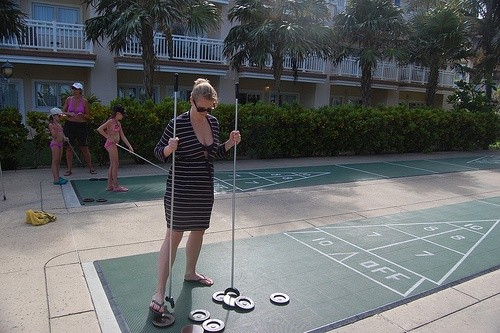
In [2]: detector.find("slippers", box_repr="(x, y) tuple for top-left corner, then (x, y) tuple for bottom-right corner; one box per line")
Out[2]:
(149, 300), (164, 314)
(184, 274), (214, 285)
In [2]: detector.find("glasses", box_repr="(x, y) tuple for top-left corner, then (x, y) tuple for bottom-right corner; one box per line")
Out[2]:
(72, 87), (79, 90)
(193, 100), (212, 113)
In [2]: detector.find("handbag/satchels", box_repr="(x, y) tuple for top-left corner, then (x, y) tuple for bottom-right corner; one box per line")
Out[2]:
(26, 209), (56, 226)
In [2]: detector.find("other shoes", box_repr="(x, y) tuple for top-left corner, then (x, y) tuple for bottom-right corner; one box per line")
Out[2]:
(59, 177), (68, 182)
(64, 171), (72, 176)
(54, 180), (66, 184)
(113, 186), (128, 191)
(90, 170), (97, 174)
(106, 186), (113, 191)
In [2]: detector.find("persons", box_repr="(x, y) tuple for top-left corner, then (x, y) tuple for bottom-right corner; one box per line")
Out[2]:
(97, 104), (133, 191)
(149, 79), (241, 315)
(48, 108), (68, 185)
(63, 82), (97, 176)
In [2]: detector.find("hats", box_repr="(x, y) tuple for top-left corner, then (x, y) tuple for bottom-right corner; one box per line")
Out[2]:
(51, 108), (65, 117)
(72, 83), (83, 90)
(113, 104), (128, 117)
